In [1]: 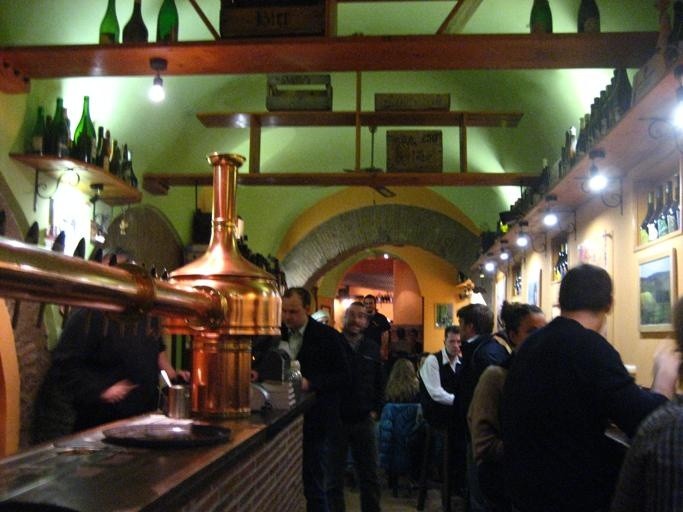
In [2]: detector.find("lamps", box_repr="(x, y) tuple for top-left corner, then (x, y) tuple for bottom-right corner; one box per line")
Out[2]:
(478, 262), (487, 281)
(582, 150), (609, 194)
(146, 57), (168, 103)
(516, 220), (530, 248)
(484, 252), (496, 273)
(542, 194), (560, 228)
(498, 238), (512, 261)
(666, 66), (682, 135)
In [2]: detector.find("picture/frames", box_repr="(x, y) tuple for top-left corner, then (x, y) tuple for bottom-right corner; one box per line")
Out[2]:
(575, 226), (615, 288)
(598, 300), (614, 347)
(432, 301), (454, 331)
(634, 246), (680, 334)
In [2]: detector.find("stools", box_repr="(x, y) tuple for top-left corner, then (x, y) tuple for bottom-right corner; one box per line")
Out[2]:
(416, 415), (468, 512)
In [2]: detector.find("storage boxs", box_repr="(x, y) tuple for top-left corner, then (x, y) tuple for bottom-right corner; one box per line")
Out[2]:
(384, 128), (445, 176)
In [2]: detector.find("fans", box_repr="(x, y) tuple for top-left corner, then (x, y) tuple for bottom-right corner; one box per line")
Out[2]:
(343, 122), (398, 198)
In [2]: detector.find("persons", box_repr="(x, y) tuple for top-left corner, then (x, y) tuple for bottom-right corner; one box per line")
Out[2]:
(609, 295), (681, 508)
(258, 285), (375, 512)
(324, 299), (388, 510)
(498, 262), (681, 510)
(456, 303), (495, 421)
(307, 293), (424, 421)
(31, 243), (161, 445)
(419, 325), (467, 498)
(465, 299), (549, 510)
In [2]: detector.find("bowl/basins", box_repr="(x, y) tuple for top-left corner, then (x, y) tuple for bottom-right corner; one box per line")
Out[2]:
(156, 382), (190, 414)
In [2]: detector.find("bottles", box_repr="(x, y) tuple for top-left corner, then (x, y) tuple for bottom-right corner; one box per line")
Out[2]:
(155, 0), (178, 43)
(167, 384), (186, 418)
(639, 172), (680, 240)
(515, 271), (521, 295)
(98, 0), (119, 44)
(528, 0), (552, 35)
(560, 67), (631, 178)
(541, 157), (550, 191)
(576, 1), (600, 34)
(554, 241), (569, 280)
(376, 294), (392, 302)
(120, 0), (148, 43)
(288, 359), (303, 403)
(31, 96), (137, 189)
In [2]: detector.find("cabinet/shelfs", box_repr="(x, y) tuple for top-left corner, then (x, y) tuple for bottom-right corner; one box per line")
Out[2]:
(139, 104), (546, 196)
